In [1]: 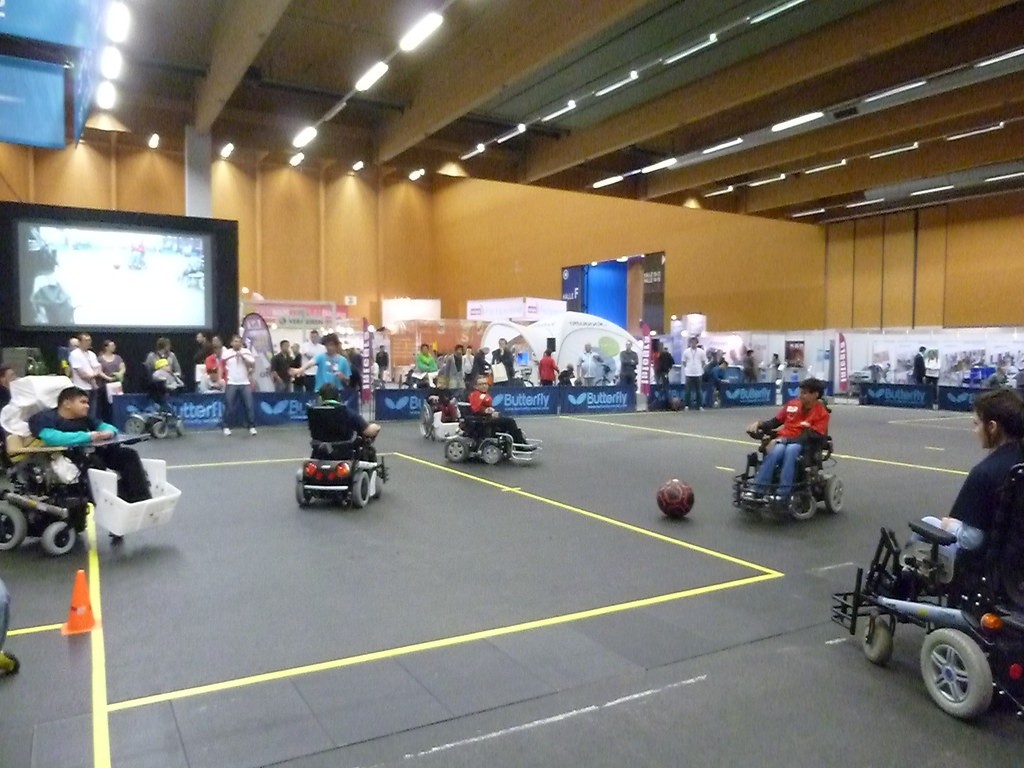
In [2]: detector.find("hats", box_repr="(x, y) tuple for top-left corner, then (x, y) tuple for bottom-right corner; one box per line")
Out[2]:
(687, 336), (699, 345)
(154, 358), (169, 369)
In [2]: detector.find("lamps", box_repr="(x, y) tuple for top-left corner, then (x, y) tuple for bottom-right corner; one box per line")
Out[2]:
(86, 0), (1024, 221)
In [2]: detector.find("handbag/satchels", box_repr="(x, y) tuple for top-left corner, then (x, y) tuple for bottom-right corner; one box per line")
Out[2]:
(491, 357), (510, 383)
(435, 374), (450, 390)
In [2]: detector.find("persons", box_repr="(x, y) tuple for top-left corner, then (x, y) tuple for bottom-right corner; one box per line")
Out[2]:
(539, 343), (604, 386)
(742, 350), (758, 383)
(987, 367), (1006, 388)
(682, 337), (729, 410)
(308, 383), (381, 477)
(194, 331), (257, 435)
(371, 346), (389, 383)
(744, 378), (828, 501)
(28, 388), (150, 500)
(771, 353), (781, 368)
(469, 375), (537, 451)
(270, 331), (363, 412)
(0, 366), (16, 444)
(148, 338), (183, 396)
(416, 338), (515, 389)
(619, 341), (638, 383)
(911, 390), (1024, 572)
(61, 334), (125, 422)
(653, 347), (675, 384)
(911, 347), (941, 402)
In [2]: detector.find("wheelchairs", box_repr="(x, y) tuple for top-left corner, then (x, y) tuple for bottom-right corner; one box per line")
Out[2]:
(295, 399), (390, 511)
(0, 376), (183, 559)
(731, 397), (847, 524)
(828, 515), (1024, 724)
(444, 404), (544, 466)
(419, 395), (473, 442)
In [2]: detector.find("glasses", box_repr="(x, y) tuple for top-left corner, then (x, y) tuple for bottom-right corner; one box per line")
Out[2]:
(206, 368), (218, 374)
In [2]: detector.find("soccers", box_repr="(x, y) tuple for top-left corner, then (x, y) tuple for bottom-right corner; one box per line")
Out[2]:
(671, 396), (680, 410)
(657, 479), (694, 516)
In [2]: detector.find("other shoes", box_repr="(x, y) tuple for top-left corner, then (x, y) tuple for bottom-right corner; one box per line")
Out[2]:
(0, 649), (20, 674)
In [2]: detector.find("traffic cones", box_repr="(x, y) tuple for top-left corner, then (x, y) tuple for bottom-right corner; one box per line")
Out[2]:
(60, 569), (96, 636)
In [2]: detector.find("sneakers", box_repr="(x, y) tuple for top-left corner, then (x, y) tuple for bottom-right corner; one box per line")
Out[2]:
(223, 428), (232, 436)
(248, 427), (258, 436)
